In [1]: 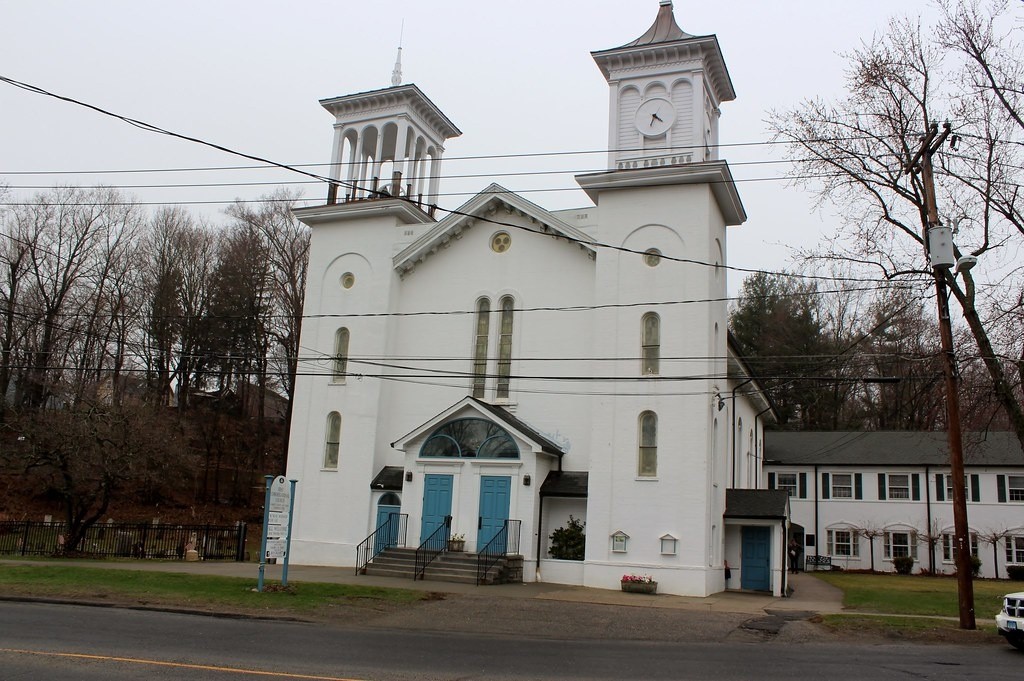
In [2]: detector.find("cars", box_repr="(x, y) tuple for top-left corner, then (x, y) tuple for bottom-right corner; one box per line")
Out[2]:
(995, 592), (1024, 650)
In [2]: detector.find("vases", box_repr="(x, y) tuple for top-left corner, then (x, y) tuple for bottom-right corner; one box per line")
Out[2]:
(448, 540), (465, 552)
(620, 580), (658, 594)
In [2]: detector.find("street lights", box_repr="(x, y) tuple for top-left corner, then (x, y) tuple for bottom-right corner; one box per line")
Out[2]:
(939, 256), (978, 628)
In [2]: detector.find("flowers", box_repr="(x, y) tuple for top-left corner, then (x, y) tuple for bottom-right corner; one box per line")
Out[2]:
(621, 573), (652, 583)
(450, 532), (465, 541)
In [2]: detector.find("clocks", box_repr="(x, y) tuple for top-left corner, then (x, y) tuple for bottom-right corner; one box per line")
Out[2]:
(633, 97), (677, 139)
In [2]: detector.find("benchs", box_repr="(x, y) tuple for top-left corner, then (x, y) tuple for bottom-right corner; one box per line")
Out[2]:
(805, 555), (832, 571)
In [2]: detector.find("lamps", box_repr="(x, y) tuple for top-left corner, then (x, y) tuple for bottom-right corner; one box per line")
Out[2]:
(522, 472), (530, 486)
(405, 469), (412, 482)
